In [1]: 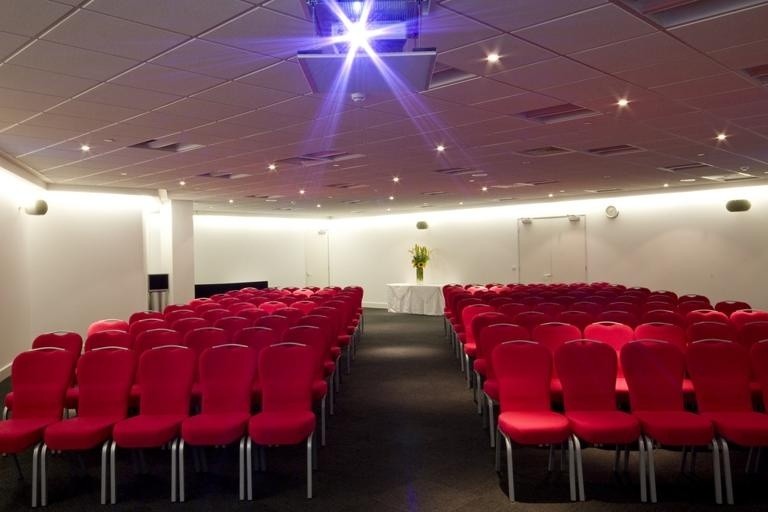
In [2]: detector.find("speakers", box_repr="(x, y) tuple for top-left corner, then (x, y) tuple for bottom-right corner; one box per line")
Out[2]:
(23, 200), (48, 216)
(725, 199), (751, 212)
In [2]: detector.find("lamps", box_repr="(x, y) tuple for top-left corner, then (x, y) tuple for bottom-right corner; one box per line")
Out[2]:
(16, 199), (49, 218)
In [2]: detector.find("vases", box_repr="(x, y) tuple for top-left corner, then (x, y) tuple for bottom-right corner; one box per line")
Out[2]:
(416, 267), (423, 284)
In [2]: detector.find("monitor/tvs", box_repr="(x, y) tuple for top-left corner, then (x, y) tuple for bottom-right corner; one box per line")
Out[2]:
(147, 273), (168, 292)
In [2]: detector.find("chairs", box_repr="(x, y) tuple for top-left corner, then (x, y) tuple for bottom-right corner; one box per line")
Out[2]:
(0, 284), (364, 510)
(438, 280), (766, 508)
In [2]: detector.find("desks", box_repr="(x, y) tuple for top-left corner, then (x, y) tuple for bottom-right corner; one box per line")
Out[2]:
(385, 282), (446, 318)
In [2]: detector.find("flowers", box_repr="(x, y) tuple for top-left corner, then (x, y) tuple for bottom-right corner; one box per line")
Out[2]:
(409, 244), (431, 268)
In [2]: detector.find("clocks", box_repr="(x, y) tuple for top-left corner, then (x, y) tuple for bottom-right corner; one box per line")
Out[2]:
(605, 205), (620, 219)
